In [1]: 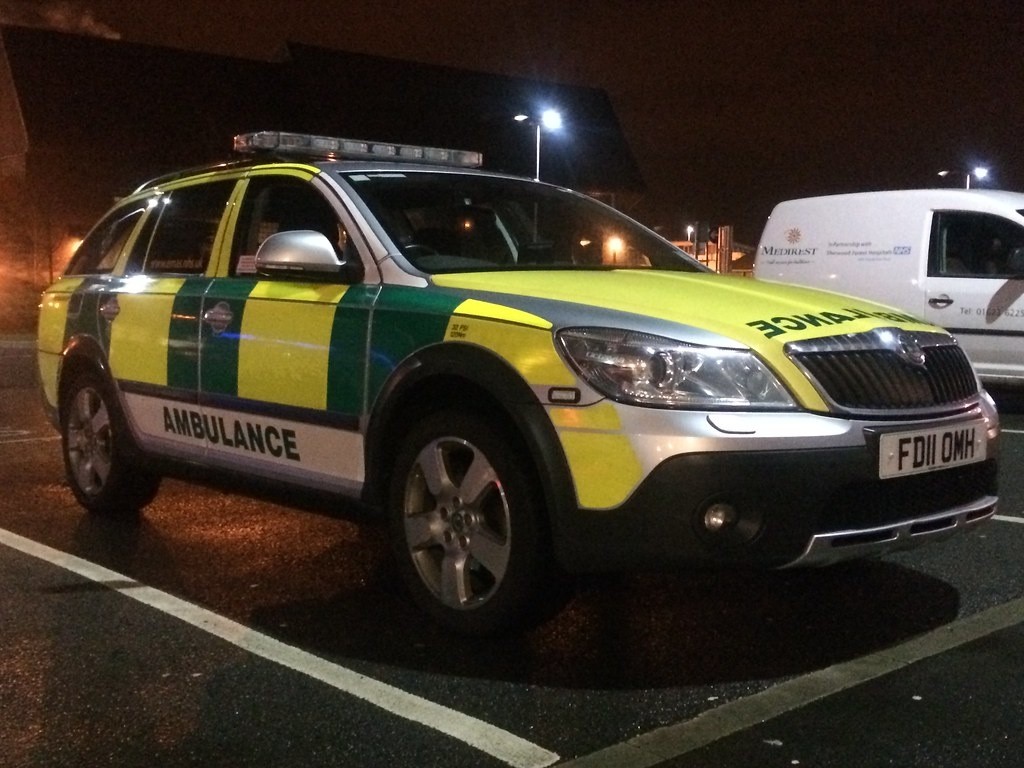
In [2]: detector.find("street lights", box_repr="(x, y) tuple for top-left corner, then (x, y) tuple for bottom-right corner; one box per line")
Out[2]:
(686, 224), (696, 240)
(963, 158), (989, 190)
(534, 105), (561, 181)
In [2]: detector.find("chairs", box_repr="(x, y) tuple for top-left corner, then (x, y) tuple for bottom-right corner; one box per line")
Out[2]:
(279, 202), (343, 263)
(413, 228), (468, 257)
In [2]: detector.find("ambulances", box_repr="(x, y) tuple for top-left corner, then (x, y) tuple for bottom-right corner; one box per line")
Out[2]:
(752, 187), (1024, 401)
(35, 131), (1002, 631)
